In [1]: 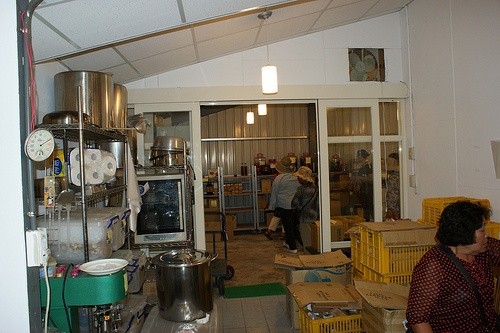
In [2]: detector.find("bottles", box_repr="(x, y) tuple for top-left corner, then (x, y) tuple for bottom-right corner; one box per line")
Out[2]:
(267, 158), (277, 175)
(287, 151), (298, 172)
(206, 181), (213, 195)
(300, 152), (318, 173)
(241, 162), (248, 176)
(254, 152), (266, 175)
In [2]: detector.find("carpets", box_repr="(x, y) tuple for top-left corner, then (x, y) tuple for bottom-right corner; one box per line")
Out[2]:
(223, 282), (286, 299)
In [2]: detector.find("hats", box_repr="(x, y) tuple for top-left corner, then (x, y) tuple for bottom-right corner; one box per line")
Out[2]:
(294, 166), (313, 183)
(275, 157), (296, 173)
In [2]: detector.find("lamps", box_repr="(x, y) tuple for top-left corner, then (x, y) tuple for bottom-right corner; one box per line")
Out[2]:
(246, 11), (278, 125)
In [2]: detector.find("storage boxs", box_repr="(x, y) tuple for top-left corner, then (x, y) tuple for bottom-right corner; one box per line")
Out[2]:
(274, 214), (500, 333)
(266, 213), (273, 226)
(258, 195), (266, 209)
(205, 215), (236, 243)
(33, 208), (146, 293)
(261, 178), (271, 193)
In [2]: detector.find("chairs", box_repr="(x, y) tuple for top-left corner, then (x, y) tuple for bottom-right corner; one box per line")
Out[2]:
(205, 210), (228, 261)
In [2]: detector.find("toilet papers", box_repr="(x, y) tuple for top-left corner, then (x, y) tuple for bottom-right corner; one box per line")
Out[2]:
(85, 148), (102, 167)
(102, 155), (117, 177)
(87, 167), (104, 184)
(69, 147), (86, 167)
(70, 167), (88, 186)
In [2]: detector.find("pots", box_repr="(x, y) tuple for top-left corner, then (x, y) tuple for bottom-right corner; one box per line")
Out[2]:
(149, 150), (189, 167)
(147, 248), (218, 322)
(150, 136), (191, 152)
(42, 110), (91, 126)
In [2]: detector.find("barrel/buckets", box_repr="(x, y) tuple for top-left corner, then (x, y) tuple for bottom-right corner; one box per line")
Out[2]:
(54, 70), (114, 130)
(114, 83), (128, 135)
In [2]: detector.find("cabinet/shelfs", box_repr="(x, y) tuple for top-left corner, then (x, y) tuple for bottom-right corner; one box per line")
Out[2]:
(203, 165), (316, 232)
(34, 84), (130, 265)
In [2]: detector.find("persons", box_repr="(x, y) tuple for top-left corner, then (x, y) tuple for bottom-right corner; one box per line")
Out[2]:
(406, 200), (500, 333)
(355, 149), (400, 222)
(269, 156), (319, 253)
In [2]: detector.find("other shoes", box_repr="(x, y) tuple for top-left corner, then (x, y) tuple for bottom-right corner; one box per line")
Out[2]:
(265, 232), (273, 239)
(288, 250), (297, 253)
(280, 234), (286, 240)
(283, 242), (289, 248)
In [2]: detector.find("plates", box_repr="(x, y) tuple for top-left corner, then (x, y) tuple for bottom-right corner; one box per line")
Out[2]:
(79, 258), (129, 275)
